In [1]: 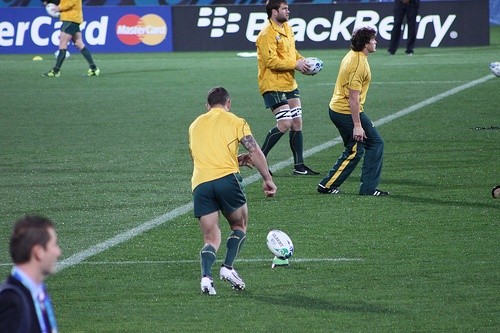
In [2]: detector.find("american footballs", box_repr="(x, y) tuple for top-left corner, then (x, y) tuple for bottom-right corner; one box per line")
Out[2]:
(298, 56), (324, 76)
(265, 229), (295, 260)
(45, 3), (61, 19)
(487, 62), (500, 77)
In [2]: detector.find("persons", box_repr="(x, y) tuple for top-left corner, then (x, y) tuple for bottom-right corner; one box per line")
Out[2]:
(388, 0), (420, 56)
(315, 27), (391, 197)
(255, 0), (321, 177)
(188, 85), (277, 296)
(43, 0), (101, 77)
(0, 214), (63, 333)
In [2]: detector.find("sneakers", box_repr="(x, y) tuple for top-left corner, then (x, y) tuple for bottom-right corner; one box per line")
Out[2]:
(293, 165), (320, 175)
(41, 69), (60, 78)
(200, 275), (217, 295)
(86, 67), (100, 76)
(317, 186), (340, 194)
(373, 189), (390, 195)
(219, 264), (245, 290)
